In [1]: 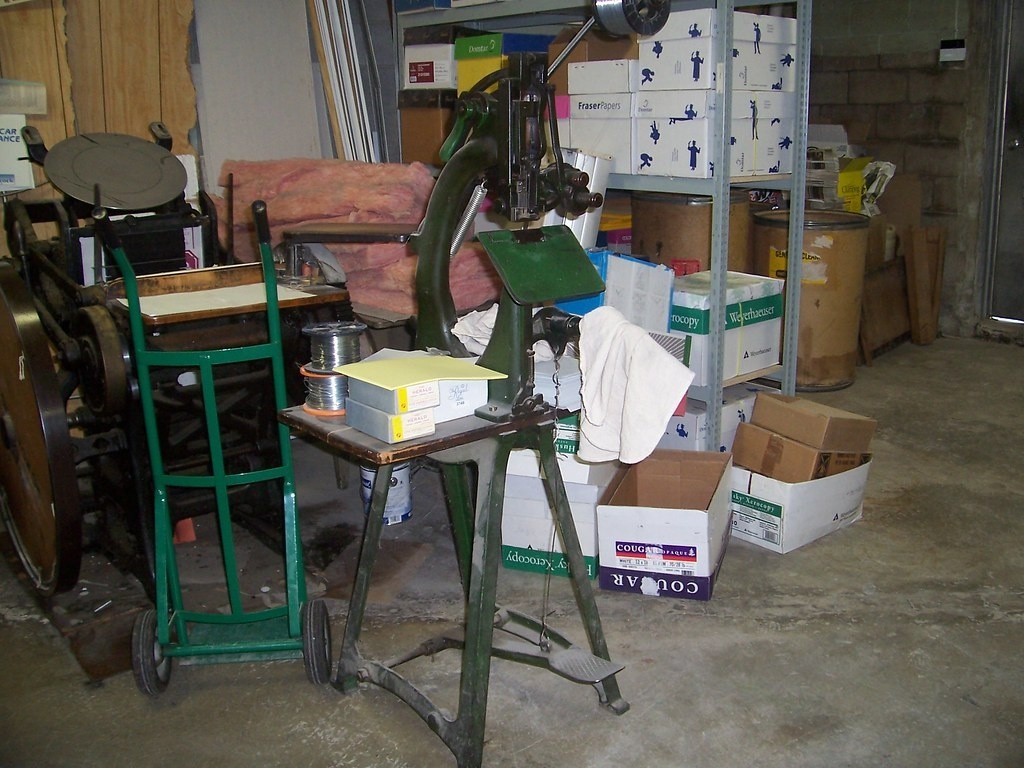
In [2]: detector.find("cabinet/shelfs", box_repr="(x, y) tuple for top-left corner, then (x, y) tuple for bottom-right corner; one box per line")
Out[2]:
(389, 0), (811, 456)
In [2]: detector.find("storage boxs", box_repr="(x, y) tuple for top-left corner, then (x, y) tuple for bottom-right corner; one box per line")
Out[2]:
(344, 0), (947, 602)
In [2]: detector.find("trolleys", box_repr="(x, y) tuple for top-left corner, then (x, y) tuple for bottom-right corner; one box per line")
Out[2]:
(88, 197), (334, 697)
